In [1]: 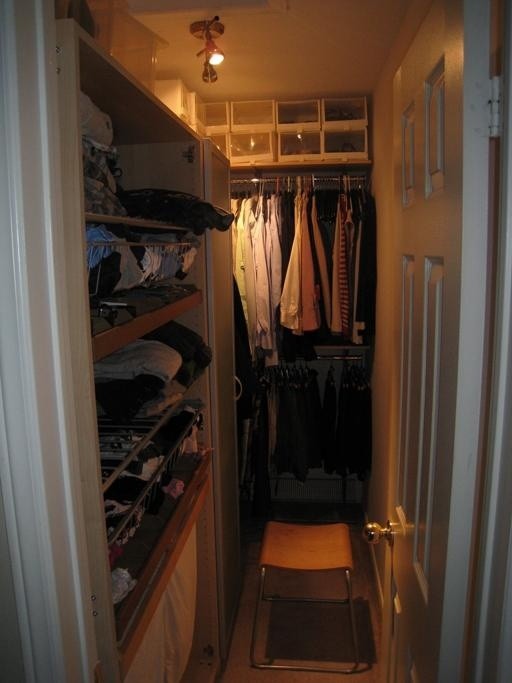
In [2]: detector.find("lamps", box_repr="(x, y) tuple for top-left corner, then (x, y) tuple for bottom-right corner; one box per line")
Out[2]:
(187, 19), (226, 83)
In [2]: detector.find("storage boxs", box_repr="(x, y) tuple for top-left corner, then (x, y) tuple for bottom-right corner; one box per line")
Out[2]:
(154, 79), (370, 160)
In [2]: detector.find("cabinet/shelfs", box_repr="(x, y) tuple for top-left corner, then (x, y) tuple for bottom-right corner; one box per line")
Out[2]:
(64, 19), (244, 683)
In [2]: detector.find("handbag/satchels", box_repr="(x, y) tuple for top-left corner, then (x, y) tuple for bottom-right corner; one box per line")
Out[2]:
(82, 136), (127, 217)
(117, 188), (234, 235)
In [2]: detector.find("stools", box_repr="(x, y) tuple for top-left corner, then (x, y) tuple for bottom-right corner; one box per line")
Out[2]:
(250, 518), (361, 674)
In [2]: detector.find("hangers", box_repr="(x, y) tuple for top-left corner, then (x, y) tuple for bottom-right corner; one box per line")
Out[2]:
(233, 174), (372, 220)
(251, 356), (370, 395)
